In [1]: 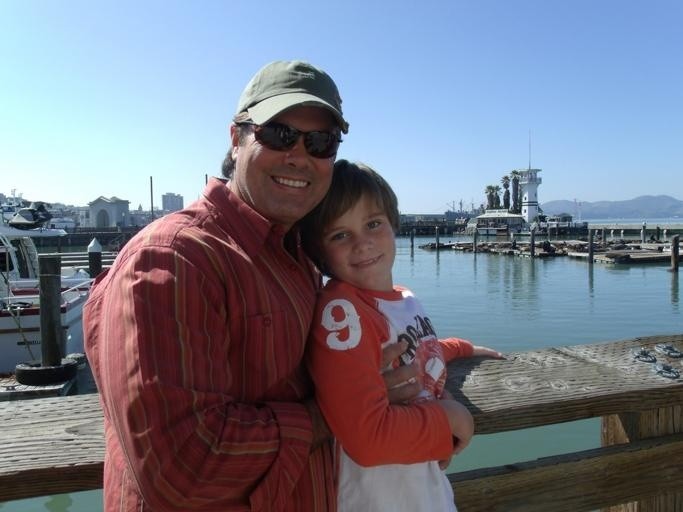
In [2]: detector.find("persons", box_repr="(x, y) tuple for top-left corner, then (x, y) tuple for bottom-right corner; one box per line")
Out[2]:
(306, 160), (499, 512)
(79, 60), (419, 512)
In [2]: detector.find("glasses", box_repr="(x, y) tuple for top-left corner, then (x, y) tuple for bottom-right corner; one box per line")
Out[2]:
(234, 117), (344, 159)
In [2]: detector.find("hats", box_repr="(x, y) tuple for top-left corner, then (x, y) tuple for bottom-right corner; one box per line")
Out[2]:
(232, 57), (351, 135)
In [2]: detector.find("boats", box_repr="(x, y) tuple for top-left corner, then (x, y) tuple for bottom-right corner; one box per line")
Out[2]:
(0, 201), (97, 378)
